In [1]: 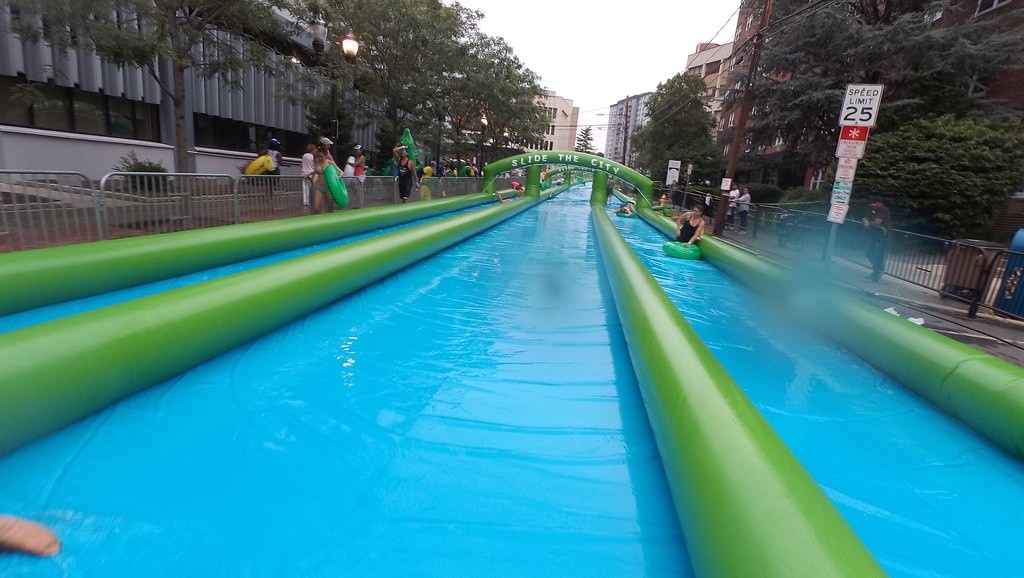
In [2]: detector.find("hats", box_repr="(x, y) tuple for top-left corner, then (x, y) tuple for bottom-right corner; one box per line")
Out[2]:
(320, 138), (333, 145)
(353, 144), (362, 150)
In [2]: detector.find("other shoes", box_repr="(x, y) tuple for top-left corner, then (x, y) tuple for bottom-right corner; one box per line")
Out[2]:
(735, 230), (746, 235)
(725, 226), (734, 230)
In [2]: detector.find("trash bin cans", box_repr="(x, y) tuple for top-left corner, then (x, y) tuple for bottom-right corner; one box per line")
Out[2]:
(940, 238), (1007, 299)
(991, 228), (1024, 321)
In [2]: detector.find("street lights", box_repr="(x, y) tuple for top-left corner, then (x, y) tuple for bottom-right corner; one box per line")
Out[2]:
(340, 28), (359, 103)
(308, 19), (329, 96)
(596, 113), (629, 166)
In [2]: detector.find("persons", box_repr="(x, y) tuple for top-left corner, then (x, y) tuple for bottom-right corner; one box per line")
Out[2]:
(540, 169), (546, 182)
(303, 138), (344, 213)
(449, 161), (455, 170)
(466, 159), (471, 165)
(511, 181), (523, 191)
(727, 184), (750, 234)
(472, 163), (478, 177)
(258, 138), (290, 202)
(607, 175), (615, 204)
(862, 196), (891, 280)
(676, 204), (705, 248)
(392, 145), (418, 202)
(613, 203), (634, 215)
(516, 171), (521, 177)
(439, 160), (447, 177)
(346, 145), (365, 209)
(669, 180), (678, 190)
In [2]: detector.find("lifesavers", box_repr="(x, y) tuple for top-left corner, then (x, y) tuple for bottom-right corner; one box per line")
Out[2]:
(663, 241), (701, 260)
(244, 155), (272, 176)
(578, 177), (582, 182)
(460, 165), (472, 176)
(343, 156), (355, 186)
(422, 166), (433, 177)
(323, 164), (349, 208)
(616, 212), (636, 218)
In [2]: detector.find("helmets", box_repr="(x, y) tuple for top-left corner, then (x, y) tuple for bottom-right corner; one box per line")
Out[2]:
(269, 138), (280, 148)
